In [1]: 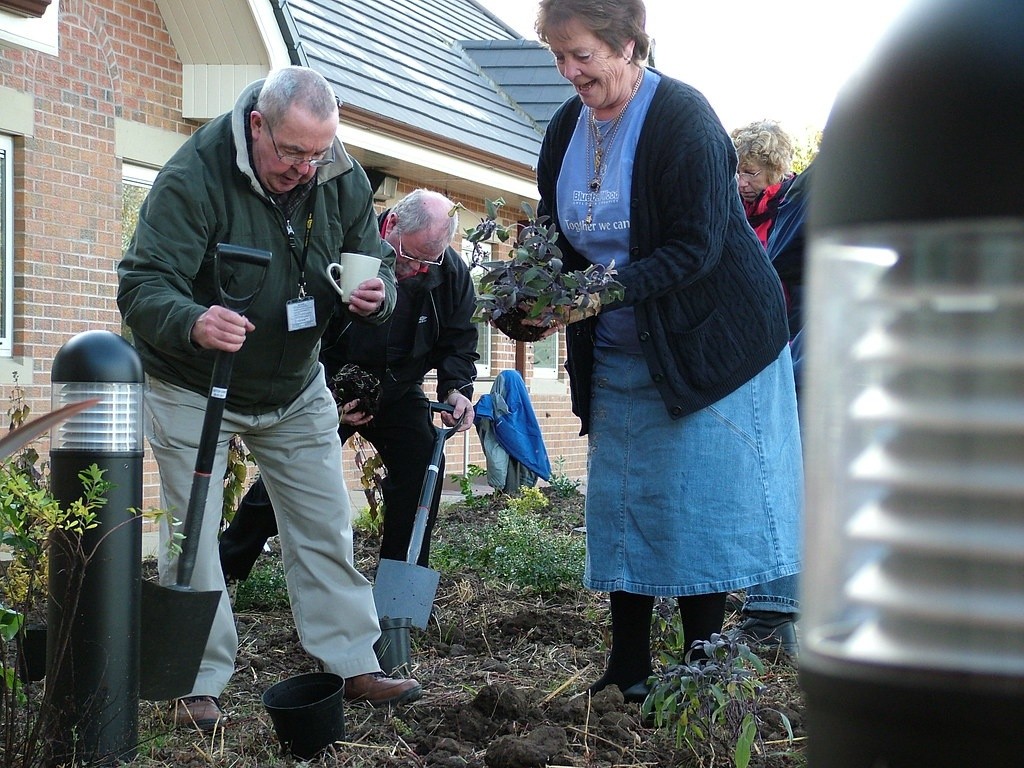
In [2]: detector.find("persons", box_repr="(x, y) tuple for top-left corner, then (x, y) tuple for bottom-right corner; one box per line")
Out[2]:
(117, 67), (424, 729)
(722, 121), (816, 660)
(490, 0), (816, 707)
(217, 188), (481, 609)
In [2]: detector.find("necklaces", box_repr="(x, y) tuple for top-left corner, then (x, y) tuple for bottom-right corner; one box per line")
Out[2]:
(585, 69), (644, 224)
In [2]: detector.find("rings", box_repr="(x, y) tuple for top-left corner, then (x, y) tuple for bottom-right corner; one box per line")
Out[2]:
(550, 320), (556, 327)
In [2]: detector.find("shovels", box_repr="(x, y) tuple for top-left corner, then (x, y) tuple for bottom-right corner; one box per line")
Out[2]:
(371, 401), (469, 633)
(134, 241), (274, 704)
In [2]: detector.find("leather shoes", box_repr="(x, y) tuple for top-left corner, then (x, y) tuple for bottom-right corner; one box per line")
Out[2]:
(344, 672), (423, 707)
(167, 696), (223, 729)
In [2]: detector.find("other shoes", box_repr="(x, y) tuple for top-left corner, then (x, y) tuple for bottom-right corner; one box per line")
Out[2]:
(222, 568), (238, 608)
(554, 676), (654, 704)
(722, 610), (798, 668)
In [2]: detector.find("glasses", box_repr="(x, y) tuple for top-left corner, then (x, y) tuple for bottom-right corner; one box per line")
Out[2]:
(736, 166), (766, 182)
(397, 225), (445, 266)
(261, 113), (336, 168)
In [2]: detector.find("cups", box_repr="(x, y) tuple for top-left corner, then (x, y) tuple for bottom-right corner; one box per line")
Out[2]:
(327, 253), (381, 304)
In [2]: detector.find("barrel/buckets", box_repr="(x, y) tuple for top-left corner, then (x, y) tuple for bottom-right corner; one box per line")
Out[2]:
(262, 673), (345, 761)
(373, 618), (412, 677)
(14, 630), (46, 683)
